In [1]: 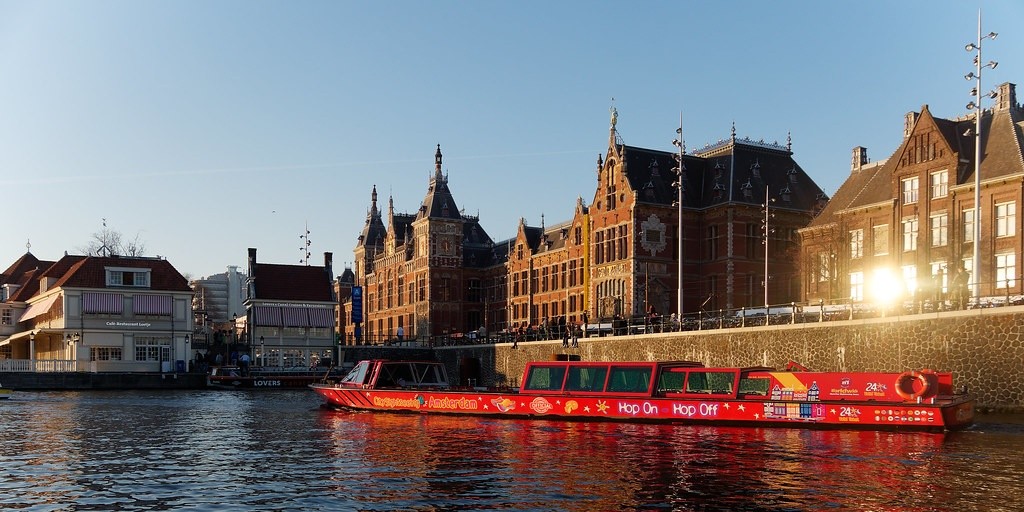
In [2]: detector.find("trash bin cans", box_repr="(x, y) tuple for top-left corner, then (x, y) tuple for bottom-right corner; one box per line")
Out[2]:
(175, 360), (185, 371)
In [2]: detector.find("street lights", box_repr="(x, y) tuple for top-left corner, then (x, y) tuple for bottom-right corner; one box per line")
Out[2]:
(761, 184), (777, 315)
(299, 220), (312, 266)
(671, 110), (687, 321)
(963, 7), (998, 308)
(505, 238), (515, 329)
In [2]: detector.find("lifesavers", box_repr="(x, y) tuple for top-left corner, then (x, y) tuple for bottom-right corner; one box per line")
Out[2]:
(894, 370), (930, 401)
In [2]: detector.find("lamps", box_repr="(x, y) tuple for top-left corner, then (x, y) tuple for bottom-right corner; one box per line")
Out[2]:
(66, 334), (71, 345)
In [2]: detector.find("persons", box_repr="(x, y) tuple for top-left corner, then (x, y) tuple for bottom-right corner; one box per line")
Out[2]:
(519, 325), (523, 335)
(397, 325), (404, 347)
(582, 310), (588, 331)
(557, 315), (565, 332)
(537, 325), (544, 341)
(526, 324), (533, 340)
(195, 350), (202, 369)
(563, 326), (578, 348)
(951, 266), (969, 310)
(216, 353), (221, 365)
(479, 324), (486, 336)
(614, 314), (627, 335)
(648, 305), (657, 333)
(240, 352), (250, 366)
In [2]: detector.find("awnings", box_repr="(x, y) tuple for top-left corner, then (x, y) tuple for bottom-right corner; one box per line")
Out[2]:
(16, 293), (60, 321)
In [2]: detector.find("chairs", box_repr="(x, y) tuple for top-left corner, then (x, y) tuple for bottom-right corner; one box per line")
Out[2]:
(410, 385), (756, 401)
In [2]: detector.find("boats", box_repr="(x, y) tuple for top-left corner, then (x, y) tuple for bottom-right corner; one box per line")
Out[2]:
(206, 366), (341, 391)
(306, 357), (978, 435)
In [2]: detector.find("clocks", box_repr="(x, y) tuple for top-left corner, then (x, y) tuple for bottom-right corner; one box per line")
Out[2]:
(439, 237), (453, 254)
